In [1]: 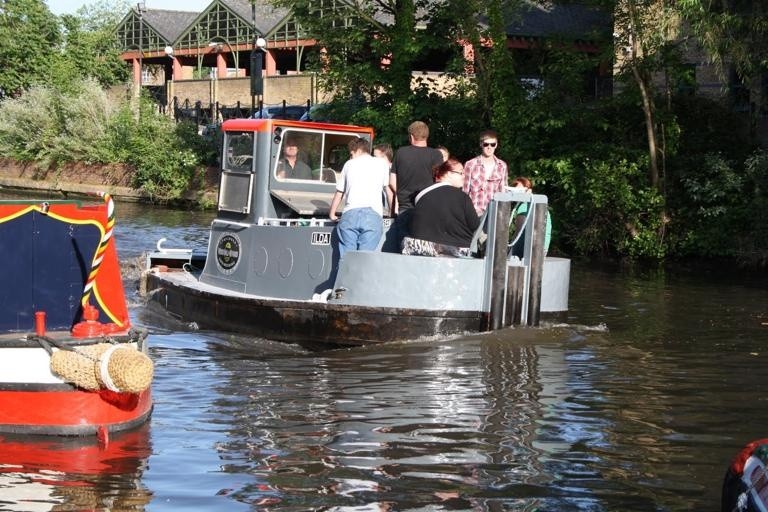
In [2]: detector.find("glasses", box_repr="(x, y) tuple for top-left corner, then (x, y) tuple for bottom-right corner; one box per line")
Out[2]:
(484, 143), (496, 147)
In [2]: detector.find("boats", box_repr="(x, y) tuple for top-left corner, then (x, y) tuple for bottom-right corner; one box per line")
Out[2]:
(0, 190), (156, 438)
(138, 118), (572, 350)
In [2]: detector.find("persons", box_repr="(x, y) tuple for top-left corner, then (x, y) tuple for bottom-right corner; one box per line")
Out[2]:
(400, 158), (479, 258)
(386, 121), (444, 253)
(461, 130), (509, 259)
(435, 146), (449, 163)
(373, 143), (399, 218)
(329, 137), (397, 257)
(276, 138), (313, 179)
(510, 177), (552, 259)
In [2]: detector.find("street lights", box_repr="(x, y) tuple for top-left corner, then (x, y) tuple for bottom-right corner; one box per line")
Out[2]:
(136, 0), (147, 96)
